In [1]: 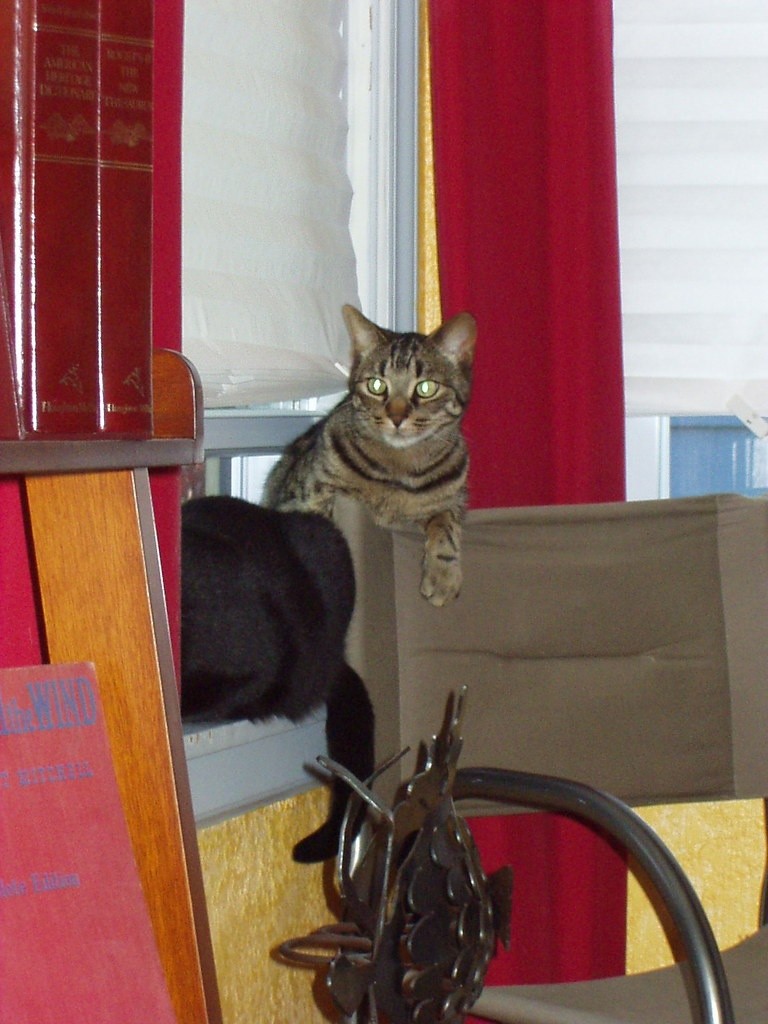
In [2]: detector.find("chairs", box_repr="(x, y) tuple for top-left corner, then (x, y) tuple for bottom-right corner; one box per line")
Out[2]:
(328, 493), (768, 1024)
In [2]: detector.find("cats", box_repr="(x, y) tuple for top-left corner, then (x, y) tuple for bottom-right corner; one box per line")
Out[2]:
(255, 302), (481, 607)
(182, 493), (380, 867)
